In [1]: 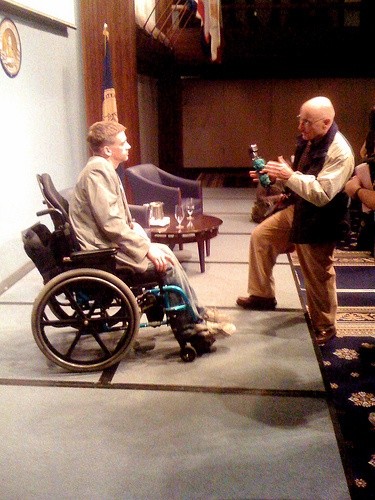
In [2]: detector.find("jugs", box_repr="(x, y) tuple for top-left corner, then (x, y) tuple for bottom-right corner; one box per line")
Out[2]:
(148, 202), (165, 222)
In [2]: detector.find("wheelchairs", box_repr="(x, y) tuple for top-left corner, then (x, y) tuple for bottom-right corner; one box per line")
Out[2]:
(20, 173), (217, 373)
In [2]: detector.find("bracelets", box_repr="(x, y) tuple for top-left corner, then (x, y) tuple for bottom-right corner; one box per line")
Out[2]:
(355, 188), (364, 198)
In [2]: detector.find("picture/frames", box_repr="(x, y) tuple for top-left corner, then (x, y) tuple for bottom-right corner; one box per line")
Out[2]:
(0, 20), (22, 77)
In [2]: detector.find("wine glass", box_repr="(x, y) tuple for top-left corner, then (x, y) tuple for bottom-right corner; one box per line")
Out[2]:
(185, 197), (195, 221)
(175, 205), (185, 229)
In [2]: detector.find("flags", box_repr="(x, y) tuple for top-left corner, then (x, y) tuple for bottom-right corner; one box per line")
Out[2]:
(102, 30), (125, 190)
(192, 0), (222, 63)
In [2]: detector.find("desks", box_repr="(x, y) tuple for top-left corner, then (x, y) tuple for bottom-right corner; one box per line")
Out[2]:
(147, 213), (222, 274)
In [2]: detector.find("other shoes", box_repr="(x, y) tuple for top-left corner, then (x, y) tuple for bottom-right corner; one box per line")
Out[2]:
(236, 294), (277, 308)
(316, 327), (337, 343)
(184, 309), (235, 337)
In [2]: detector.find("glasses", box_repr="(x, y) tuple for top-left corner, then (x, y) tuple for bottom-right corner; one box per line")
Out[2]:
(297, 115), (327, 127)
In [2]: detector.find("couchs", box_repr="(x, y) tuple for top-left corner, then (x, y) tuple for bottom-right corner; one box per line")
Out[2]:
(126, 164), (203, 249)
(60, 186), (151, 242)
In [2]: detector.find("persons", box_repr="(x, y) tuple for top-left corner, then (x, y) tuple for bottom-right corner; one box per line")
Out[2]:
(236, 96), (355, 347)
(69, 122), (238, 340)
(345, 109), (375, 257)
